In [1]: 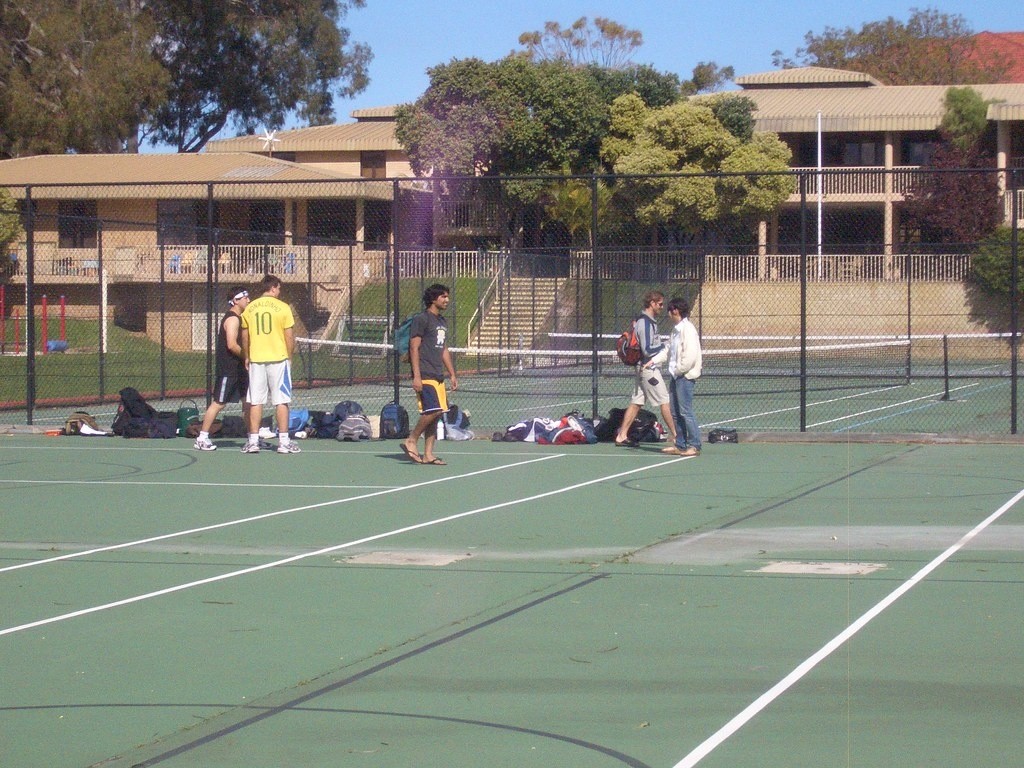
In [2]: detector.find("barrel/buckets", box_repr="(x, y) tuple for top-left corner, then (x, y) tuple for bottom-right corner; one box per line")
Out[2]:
(177, 399), (199, 438)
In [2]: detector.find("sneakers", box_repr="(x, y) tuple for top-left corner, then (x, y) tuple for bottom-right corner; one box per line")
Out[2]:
(247, 427), (276, 440)
(277, 441), (301, 454)
(260, 440), (274, 449)
(240, 442), (260, 453)
(192, 439), (217, 450)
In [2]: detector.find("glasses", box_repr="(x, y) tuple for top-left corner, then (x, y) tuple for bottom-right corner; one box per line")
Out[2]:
(667, 307), (672, 311)
(655, 302), (662, 306)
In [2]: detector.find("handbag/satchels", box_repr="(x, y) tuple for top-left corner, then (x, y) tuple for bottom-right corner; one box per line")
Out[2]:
(45, 390), (273, 442)
(593, 407), (667, 443)
(708, 428), (738, 444)
(421, 402), (476, 441)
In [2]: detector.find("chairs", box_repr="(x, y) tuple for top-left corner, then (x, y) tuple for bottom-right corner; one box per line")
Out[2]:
(169, 252), (298, 274)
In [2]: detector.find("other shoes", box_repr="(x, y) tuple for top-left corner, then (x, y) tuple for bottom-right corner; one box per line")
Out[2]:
(665, 446), (687, 454)
(681, 446), (700, 456)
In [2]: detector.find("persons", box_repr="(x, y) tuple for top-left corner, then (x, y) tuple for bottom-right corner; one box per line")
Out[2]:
(241, 275), (301, 453)
(615, 290), (677, 447)
(193, 286), (273, 450)
(400, 285), (459, 465)
(643, 297), (702, 456)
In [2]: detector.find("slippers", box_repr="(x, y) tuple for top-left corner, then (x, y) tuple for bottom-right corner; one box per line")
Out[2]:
(422, 456), (447, 465)
(615, 437), (641, 447)
(399, 444), (423, 464)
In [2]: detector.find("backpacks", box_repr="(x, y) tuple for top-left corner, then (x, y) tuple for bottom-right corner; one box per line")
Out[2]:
(394, 314), (416, 363)
(617, 320), (644, 366)
(275, 399), (372, 443)
(379, 400), (409, 439)
